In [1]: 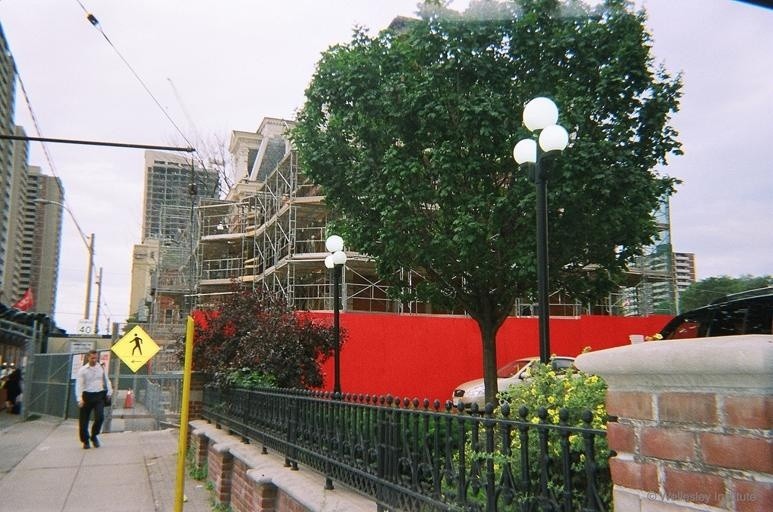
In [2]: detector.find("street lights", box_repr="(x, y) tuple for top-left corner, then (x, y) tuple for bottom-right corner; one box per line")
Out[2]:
(322, 232), (347, 398)
(510, 96), (568, 368)
(29, 198), (112, 337)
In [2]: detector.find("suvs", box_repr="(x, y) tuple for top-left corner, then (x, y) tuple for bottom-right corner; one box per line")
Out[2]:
(654, 281), (772, 340)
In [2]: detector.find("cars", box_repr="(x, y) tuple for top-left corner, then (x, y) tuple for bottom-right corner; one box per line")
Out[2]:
(451, 352), (577, 415)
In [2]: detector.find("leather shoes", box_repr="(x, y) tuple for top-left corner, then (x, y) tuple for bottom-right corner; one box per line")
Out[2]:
(83, 435), (100, 449)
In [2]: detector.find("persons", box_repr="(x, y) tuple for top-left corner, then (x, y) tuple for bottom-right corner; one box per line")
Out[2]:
(75, 349), (114, 449)
(0, 361), (23, 415)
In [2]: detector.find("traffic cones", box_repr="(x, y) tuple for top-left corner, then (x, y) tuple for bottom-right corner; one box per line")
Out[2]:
(122, 389), (136, 409)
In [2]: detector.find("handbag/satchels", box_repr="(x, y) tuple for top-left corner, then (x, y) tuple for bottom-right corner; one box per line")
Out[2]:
(104, 395), (112, 407)
